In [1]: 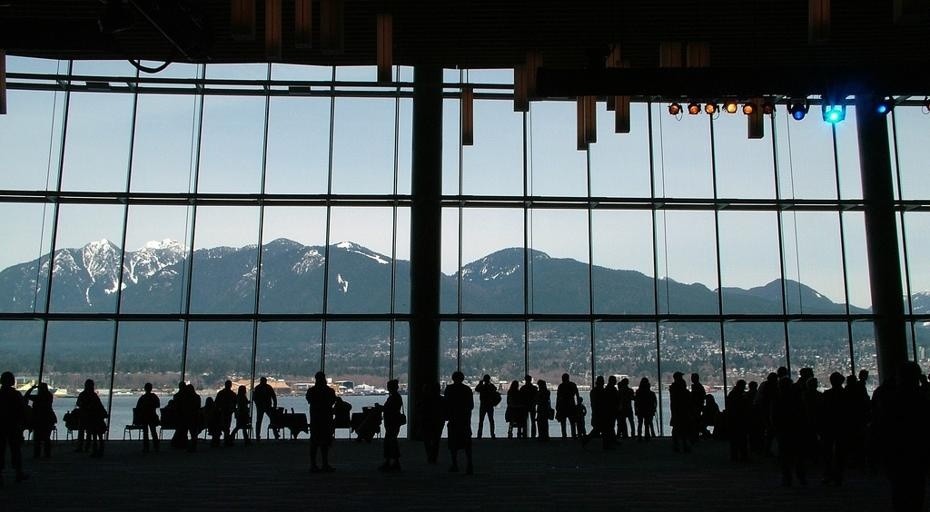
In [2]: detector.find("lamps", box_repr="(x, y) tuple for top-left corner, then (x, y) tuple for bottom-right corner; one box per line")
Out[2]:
(664, 96), (897, 127)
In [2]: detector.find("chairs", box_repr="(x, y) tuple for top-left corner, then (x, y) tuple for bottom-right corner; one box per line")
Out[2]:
(25, 409), (387, 441)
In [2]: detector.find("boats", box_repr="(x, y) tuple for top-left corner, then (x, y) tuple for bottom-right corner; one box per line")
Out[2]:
(4, 379), (720, 398)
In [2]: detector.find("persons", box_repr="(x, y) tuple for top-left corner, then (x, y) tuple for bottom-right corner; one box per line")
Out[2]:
(443, 371), (474, 475)
(669, 361), (930, 511)
(77, 379), (109, 452)
(383, 379), (402, 467)
(475, 374), (657, 451)
(1, 372), (58, 467)
(305, 372), (337, 473)
(136, 377), (280, 451)
